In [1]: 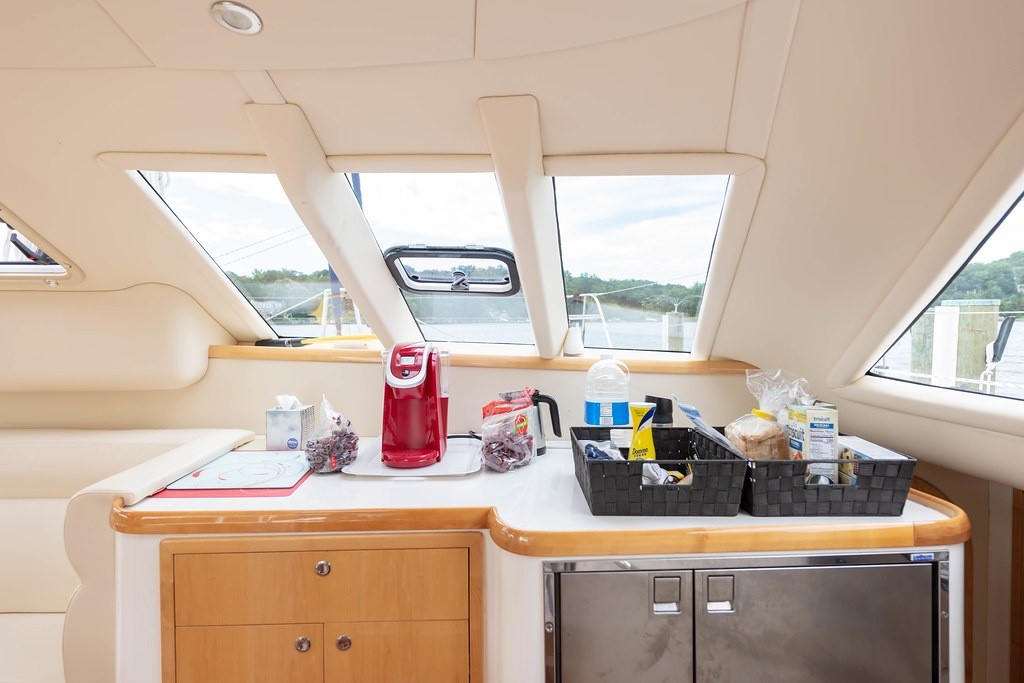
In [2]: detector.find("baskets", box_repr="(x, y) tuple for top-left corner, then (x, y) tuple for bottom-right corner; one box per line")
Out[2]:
(570, 427), (748, 516)
(714, 426), (917, 517)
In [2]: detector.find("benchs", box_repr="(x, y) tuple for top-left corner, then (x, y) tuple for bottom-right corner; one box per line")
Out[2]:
(0, 425), (253, 682)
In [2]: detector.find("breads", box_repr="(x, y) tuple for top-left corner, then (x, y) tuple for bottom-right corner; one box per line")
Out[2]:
(724, 422), (789, 460)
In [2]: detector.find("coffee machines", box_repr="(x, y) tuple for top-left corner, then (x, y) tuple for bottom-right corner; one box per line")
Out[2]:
(380, 339), (449, 469)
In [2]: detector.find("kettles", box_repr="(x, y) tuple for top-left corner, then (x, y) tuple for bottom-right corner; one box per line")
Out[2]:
(499, 389), (562, 456)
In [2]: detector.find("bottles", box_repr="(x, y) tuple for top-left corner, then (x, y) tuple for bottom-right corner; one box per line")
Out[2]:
(582, 353), (634, 428)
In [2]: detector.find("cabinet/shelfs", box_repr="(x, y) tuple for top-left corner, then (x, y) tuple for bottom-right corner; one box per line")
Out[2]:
(160, 531), (481, 683)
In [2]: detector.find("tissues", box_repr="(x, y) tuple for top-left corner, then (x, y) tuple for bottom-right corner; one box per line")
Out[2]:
(265, 394), (314, 451)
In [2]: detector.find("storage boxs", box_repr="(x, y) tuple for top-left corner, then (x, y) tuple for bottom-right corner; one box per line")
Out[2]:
(714, 426), (917, 516)
(777, 404), (839, 484)
(837, 436), (907, 484)
(569, 426), (748, 517)
